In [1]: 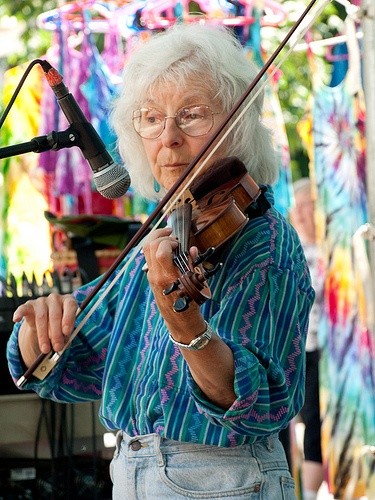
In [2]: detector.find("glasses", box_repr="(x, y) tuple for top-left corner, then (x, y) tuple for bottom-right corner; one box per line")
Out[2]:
(132, 102), (222, 140)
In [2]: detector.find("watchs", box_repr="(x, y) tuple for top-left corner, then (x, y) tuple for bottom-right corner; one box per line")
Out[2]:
(164, 319), (212, 352)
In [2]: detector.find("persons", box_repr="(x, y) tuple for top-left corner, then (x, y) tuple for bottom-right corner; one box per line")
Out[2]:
(6, 16), (317, 500)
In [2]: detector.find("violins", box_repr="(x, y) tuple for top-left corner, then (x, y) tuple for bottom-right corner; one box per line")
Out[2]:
(162, 155), (260, 312)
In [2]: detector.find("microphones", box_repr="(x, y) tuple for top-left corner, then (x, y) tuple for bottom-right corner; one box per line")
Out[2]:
(39, 59), (131, 198)
